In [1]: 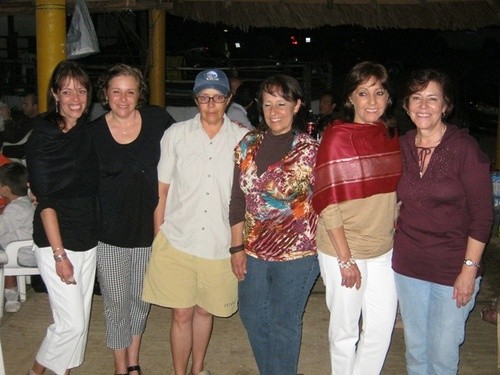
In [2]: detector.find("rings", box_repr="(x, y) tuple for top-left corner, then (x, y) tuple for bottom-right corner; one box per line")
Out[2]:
(61, 279), (66, 282)
(30, 199), (34, 203)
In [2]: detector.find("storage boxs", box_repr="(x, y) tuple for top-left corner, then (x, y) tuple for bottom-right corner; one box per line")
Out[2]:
(166, 55), (187, 80)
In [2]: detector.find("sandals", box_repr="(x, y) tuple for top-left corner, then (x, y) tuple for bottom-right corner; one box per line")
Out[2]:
(127, 365), (142, 375)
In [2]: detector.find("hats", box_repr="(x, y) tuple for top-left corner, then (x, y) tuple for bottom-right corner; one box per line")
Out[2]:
(193, 69), (230, 96)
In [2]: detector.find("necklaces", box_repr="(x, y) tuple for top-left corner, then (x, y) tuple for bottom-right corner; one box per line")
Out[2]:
(111, 109), (136, 134)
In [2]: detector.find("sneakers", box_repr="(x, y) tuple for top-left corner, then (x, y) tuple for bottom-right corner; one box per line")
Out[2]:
(4, 286), (21, 313)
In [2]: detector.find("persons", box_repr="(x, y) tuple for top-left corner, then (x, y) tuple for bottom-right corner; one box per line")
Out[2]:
(27, 60), (98, 375)
(312, 62), (403, 375)
(26, 63), (177, 375)
(228, 74), (320, 375)
(140, 69), (252, 375)
(391, 70), (495, 375)
(0, 81), (345, 312)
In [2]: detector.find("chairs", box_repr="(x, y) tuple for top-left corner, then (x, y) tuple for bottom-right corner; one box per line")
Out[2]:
(0, 239), (40, 318)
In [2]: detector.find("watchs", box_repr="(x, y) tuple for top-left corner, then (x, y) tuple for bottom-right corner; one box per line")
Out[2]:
(463, 259), (480, 268)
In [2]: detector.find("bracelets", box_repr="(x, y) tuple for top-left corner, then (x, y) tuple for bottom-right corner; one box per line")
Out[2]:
(229, 245), (245, 254)
(53, 246), (68, 262)
(337, 253), (355, 268)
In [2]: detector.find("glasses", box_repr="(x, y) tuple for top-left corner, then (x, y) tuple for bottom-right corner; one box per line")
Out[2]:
(195, 95), (227, 104)
